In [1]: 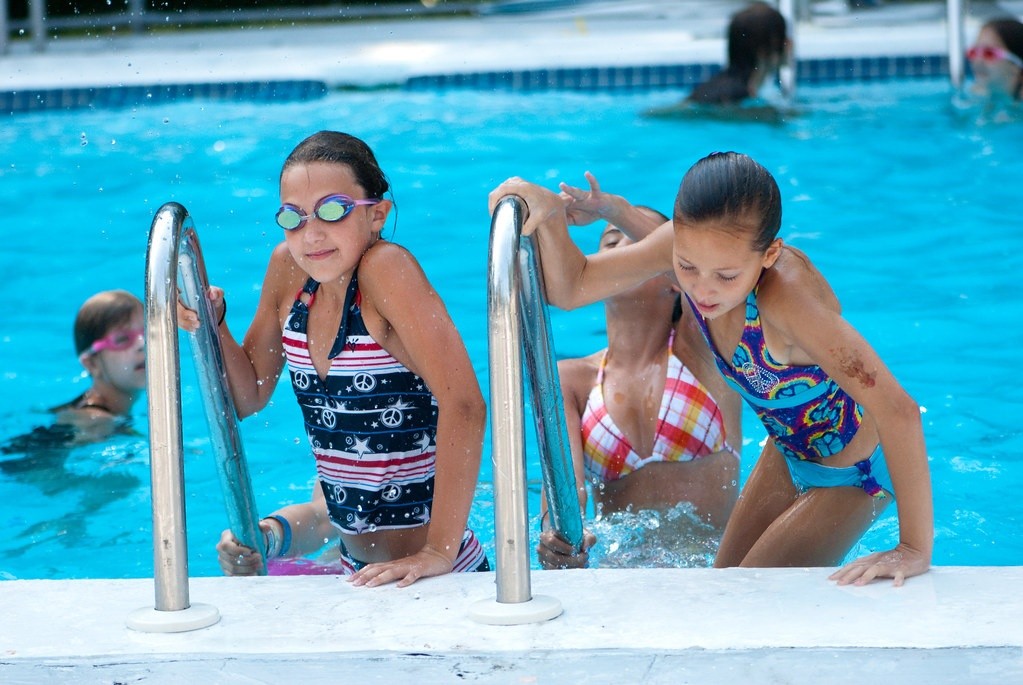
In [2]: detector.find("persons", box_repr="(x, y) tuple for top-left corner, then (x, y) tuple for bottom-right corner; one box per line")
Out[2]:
(57, 292), (147, 445)
(963, 19), (1022, 102)
(530, 170), (742, 569)
(216, 470), (342, 576)
(170, 129), (486, 590)
(681, 4), (792, 121)
(488, 151), (934, 587)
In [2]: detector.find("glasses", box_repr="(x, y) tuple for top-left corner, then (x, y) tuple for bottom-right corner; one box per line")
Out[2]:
(272, 194), (380, 231)
(85, 328), (138, 358)
(969, 43), (1022, 70)
